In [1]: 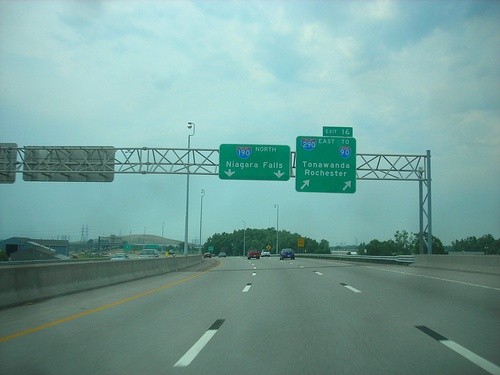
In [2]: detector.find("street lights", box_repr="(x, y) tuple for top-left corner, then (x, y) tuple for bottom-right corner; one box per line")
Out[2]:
(273, 204), (279, 254)
(183, 121), (195, 254)
(199, 188), (205, 253)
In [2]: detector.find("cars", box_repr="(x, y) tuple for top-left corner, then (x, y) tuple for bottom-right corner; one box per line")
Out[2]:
(246, 248), (260, 259)
(279, 247), (295, 260)
(260, 249), (271, 257)
(110, 252), (129, 261)
(218, 251), (226, 257)
(212, 253), (216, 257)
(203, 252), (212, 258)
(138, 248), (160, 259)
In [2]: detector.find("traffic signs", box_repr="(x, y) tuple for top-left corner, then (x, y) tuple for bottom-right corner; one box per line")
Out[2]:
(218, 143), (291, 181)
(295, 135), (357, 194)
